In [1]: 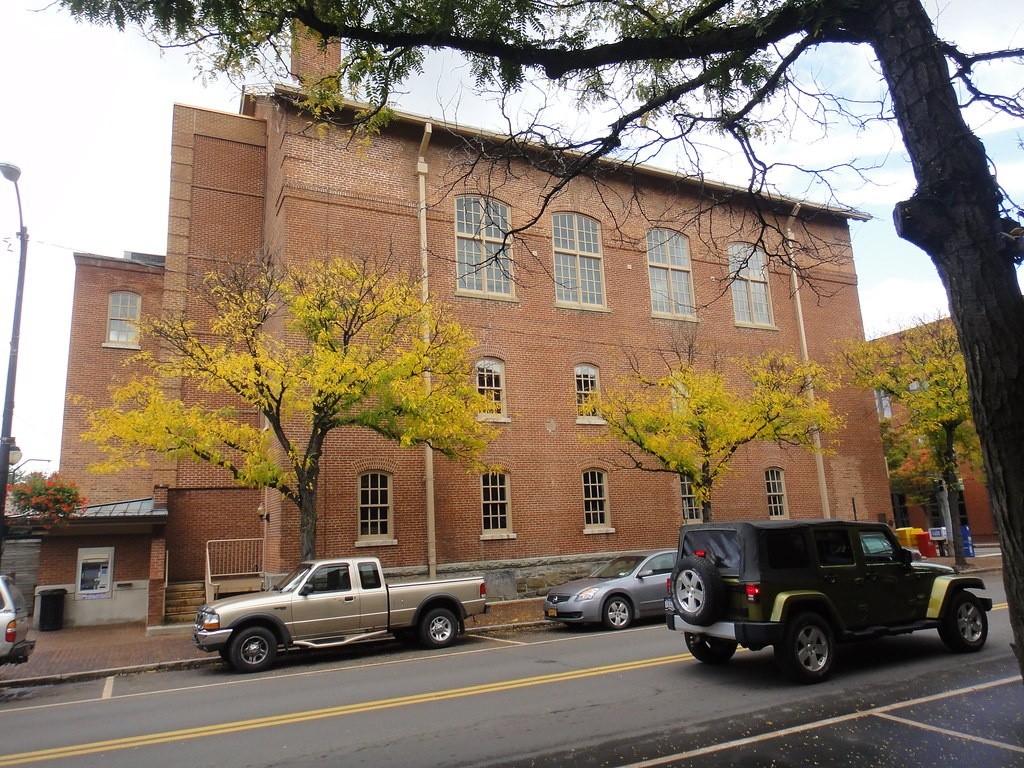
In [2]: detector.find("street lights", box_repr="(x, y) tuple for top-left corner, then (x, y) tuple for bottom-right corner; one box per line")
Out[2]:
(0, 162), (30, 561)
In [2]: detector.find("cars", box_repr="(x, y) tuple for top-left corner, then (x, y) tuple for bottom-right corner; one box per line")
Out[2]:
(0, 575), (36, 666)
(543, 548), (678, 631)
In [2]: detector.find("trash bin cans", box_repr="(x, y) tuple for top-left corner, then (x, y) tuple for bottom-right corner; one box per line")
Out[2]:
(916, 532), (937, 557)
(38, 589), (67, 631)
(896, 527), (913, 548)
(910, 528), (924, 545)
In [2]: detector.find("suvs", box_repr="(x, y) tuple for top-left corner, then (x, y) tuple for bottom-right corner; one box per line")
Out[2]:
(665, 519), (993, 684)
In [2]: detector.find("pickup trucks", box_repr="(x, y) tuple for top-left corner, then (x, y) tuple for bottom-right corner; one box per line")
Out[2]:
(193, 557), (491, 675)
